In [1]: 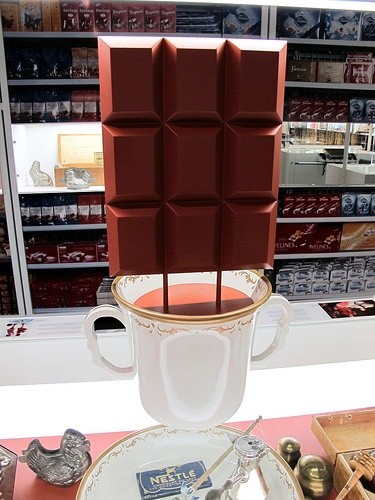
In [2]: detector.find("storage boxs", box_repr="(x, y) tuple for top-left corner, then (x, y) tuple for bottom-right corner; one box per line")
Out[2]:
(310, 410), (375, 500)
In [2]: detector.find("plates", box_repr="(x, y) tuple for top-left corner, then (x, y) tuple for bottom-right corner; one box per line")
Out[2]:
(75, 423), (304, 500)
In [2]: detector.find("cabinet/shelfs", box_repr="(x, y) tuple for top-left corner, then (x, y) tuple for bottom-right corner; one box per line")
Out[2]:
(0, 0), (374, 317)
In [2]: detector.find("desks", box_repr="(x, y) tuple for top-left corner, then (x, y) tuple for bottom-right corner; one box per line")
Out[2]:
(0, 319), (375, 500)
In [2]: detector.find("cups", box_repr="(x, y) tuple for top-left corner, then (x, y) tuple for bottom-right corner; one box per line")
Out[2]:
(84, 269), (292, 429)
